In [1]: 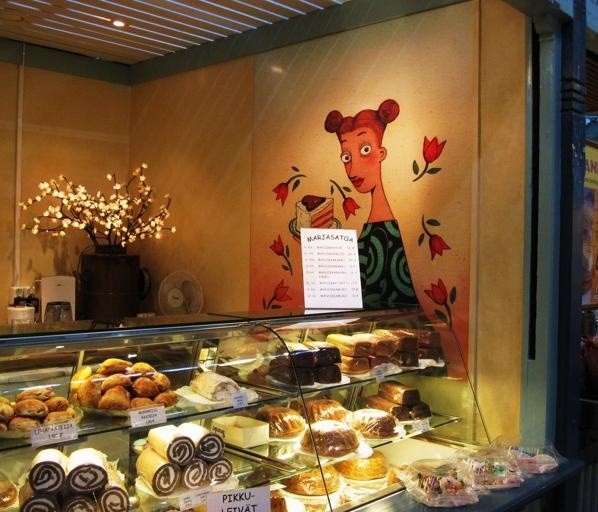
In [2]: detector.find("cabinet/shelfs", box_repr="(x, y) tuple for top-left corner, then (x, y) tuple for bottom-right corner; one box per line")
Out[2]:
(0, 303), (590, 511)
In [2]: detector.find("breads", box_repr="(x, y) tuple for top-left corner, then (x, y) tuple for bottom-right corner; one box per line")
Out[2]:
(352, 333), (396, 358)
(267, 361), (316, 386)
(318, 366), (343, 384)
(396, 352), (420, 369)
(276, 345), (316, 371)
(373, 328), (418, 352)
(342, 409), (400, 440)
(294, 422), (359, 458)
(340, 355), (370, 375)
(257, 407), (305, 440)
(376, 382), (422, 407)
(403, 328), (442, 348)
(365, 353), (397, 372)
(365, 394), (408, 421)
(416, 347), (442, 364)
(326, 334), (372, 358)
(407, 402), (432, 421)
(0, 386), (76, 436)
(72, 357), (179, 413)
(334, 450), (390, 480)
(303, 342), (343, 365)
(304, 396), (344, 423)
(285, 467), (339, 497)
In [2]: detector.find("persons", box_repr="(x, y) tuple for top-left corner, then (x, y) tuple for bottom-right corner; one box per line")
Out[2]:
(324, 98), (449, 378)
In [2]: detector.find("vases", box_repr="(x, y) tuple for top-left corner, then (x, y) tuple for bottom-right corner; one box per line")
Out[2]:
(79, 243), (144, 322)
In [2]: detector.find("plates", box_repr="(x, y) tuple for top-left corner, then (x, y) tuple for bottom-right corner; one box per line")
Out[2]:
(361, 422), (406, 440)
(265, 373), (351, 388)
(135, 472), (240, 499)
(296, 441), (373, 459)
(397, 416), (432, 426)
(0, 405), (84, 438)
(349, 364), (402, 380)
(80, 403), (177, 417)
(269, 416), (309, 442)
(401, 358), (445, 371)
(176, 386), (259, 404)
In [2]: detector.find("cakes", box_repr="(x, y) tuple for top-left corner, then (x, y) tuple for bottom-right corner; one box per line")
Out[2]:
(148, 425), (194, 465)
(459, 455), (523, 490)
(135, 449), (181, 497)
(402, 463), (477, 507)
(62, 492), (97, 512)
(67, 446), (109, 493)
(206, 458), (233, 485)
(18, 484), (60, 512)
(100, 467), (129, 512)
(181, 458), (208, 490)
(179, 422), (224, 462)
(191, 370), (241, 403)
(28, 448), (68, 496)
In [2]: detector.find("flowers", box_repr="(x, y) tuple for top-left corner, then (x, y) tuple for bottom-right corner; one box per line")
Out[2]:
(18, 161), (177, 247)
